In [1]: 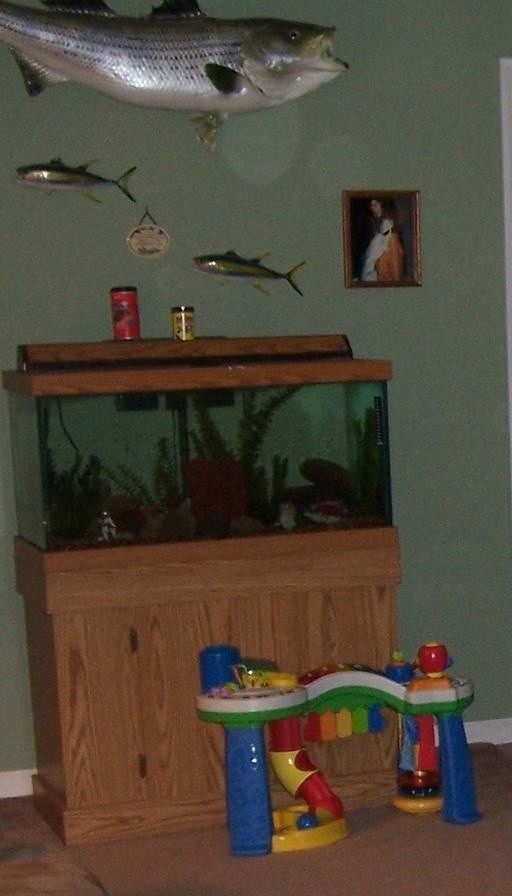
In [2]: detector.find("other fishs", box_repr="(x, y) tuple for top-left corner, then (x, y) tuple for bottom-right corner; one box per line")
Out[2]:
(13, 156), (140, 212)
(186, 249), (308, 306)
(0, 0), (351, 117)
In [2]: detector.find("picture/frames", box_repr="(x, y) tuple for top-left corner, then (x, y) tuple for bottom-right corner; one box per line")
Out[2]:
(341, 188), (422, 288)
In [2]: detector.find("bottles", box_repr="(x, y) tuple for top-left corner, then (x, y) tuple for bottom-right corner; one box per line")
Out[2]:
(109, 286), (142, 341)
(172, 305), (195, 341)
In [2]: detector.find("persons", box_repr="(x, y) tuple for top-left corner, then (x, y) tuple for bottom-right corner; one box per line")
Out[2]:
(358, 196), (410, 282)
